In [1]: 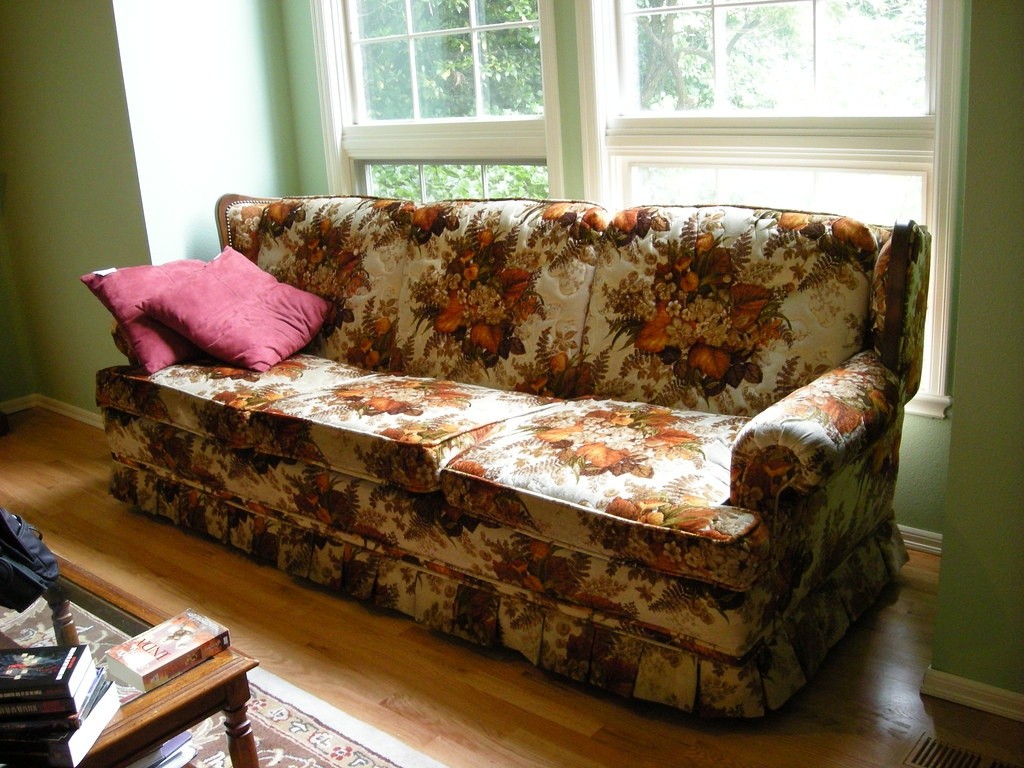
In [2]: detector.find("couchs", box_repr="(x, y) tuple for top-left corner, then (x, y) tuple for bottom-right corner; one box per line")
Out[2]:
(94, 193), (931, 724)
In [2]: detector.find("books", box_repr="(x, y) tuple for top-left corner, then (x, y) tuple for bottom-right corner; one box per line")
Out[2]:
(0, 644), (198, 768)
(105, 608), (231, 693)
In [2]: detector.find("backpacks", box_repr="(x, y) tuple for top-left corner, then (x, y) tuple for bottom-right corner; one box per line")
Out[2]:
(0, 507), (59, 613)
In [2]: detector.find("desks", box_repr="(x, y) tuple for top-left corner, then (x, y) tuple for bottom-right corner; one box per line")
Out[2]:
(0, 552), (260, 768)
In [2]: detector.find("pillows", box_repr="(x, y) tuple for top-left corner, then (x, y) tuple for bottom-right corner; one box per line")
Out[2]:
(81, 259), (209, 375)
(135, 245), (334, 372)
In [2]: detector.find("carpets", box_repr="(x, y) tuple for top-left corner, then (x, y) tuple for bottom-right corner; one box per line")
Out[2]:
(188, 666), (451, 768)
